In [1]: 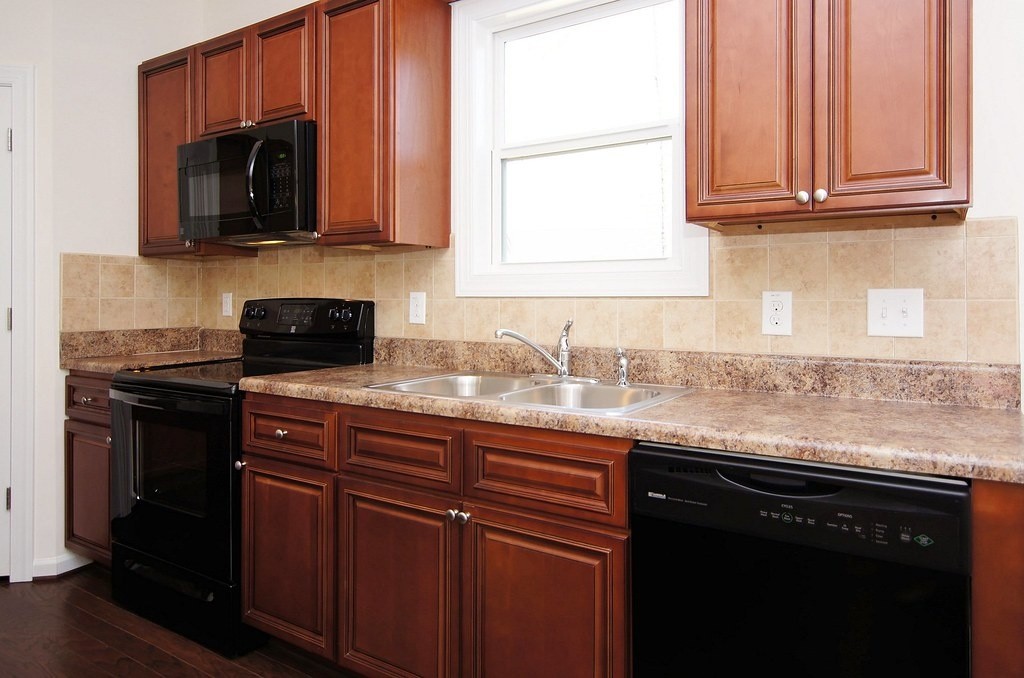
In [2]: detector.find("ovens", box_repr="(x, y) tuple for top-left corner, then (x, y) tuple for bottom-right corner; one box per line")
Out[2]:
(104, 388), (242, 656)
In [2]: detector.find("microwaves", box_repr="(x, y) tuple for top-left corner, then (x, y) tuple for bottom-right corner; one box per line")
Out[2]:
(178, 121), (315, 248)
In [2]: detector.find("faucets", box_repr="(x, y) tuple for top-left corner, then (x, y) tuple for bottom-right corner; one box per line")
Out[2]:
(494, 317), (575, 376)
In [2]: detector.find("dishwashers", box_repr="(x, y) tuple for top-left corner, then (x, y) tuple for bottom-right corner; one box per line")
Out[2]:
(626, 442), (973, 678)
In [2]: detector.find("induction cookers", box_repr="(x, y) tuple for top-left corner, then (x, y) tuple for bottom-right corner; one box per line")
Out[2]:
(113, 298), (375, 393)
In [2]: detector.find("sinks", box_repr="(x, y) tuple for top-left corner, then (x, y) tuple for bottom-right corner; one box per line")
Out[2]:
(498, 383), (663, 410)
(393, 374), (556, 397)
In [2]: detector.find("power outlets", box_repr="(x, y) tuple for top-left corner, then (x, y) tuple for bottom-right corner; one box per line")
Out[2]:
(867, 288), (923, 338)
(222, 292), (232, 317)
(409, 292), (426, 324)
(761, 291), (793, 336)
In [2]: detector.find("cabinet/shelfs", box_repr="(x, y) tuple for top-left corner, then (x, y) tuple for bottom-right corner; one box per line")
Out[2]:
(63, 375), (113, 567)
(337, 416), (630, 678)
(685, 0), (969, 220)
(194, 3), (317, 141)
(317, 0), (394, 246)
(234, 400), (341, 663)
(138, 50), (201, 257)
